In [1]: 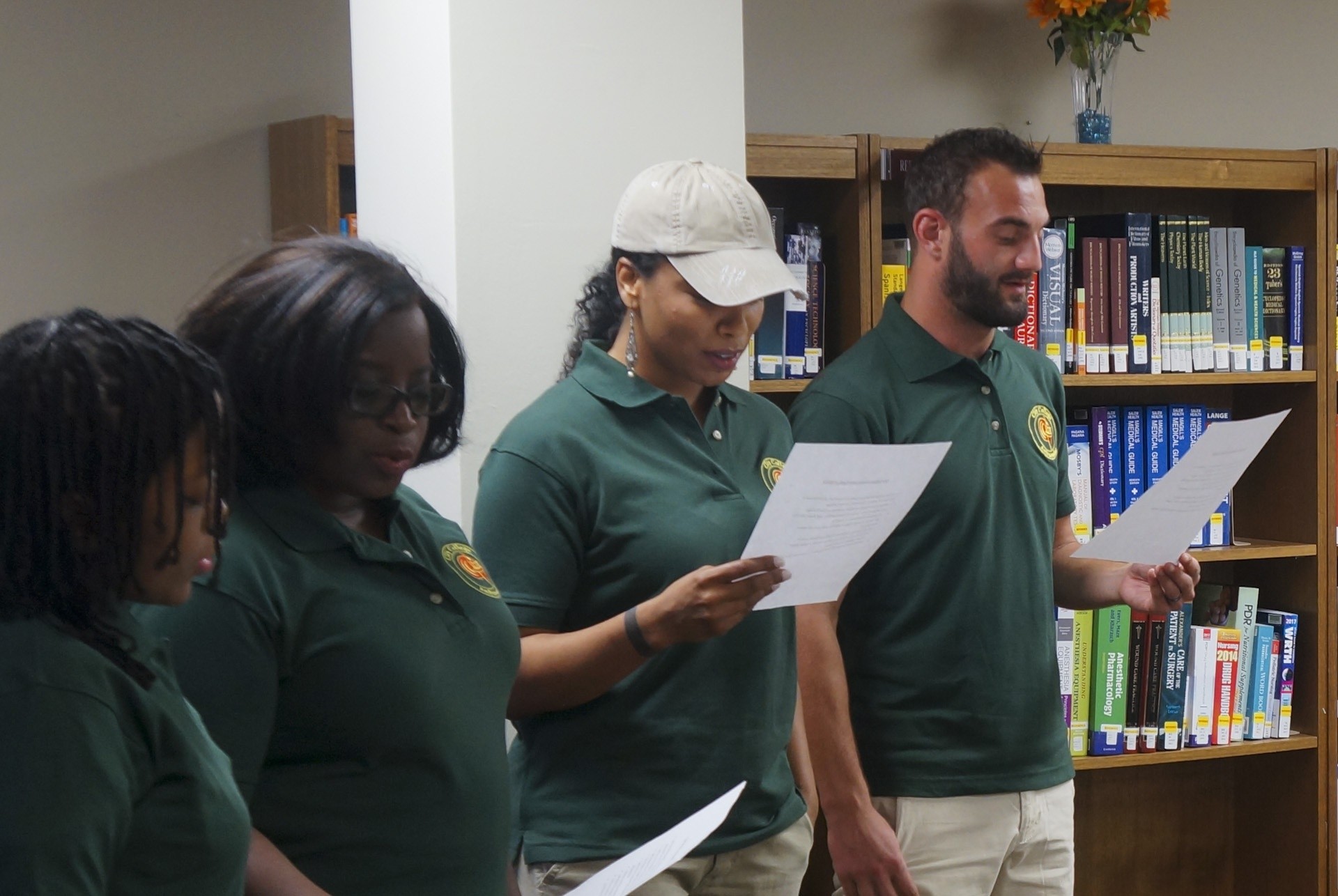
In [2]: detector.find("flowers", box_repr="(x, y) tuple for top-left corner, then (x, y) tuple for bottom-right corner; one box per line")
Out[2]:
(1024, 0), (1174, 75)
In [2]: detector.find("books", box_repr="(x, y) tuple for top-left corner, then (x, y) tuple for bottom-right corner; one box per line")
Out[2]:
(1067, 401), (1233, 549)
(881, 208), (1308, 374)
(1051, 582), (1300, 758)
(748, 206), (827, 380)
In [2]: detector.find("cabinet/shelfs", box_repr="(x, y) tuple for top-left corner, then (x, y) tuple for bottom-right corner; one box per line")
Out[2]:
(266, 116), (1338, 896)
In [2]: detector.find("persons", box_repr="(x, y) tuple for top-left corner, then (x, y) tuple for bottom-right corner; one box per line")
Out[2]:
(788, 125), (1203, 896)
(120, 234), (524, 896)
(0, 307), (334, 896)
(469, 158), (824, 896)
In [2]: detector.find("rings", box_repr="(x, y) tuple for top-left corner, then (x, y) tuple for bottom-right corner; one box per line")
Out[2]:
(1164, 592), (1181, 602)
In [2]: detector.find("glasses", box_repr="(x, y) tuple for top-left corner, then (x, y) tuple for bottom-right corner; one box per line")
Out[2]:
(350, 368), (454, 422)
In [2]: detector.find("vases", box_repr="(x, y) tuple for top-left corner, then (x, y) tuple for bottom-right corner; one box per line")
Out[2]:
(1066, 27), (1130, 143)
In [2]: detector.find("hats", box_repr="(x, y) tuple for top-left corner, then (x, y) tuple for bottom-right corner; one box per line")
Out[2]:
(612, 157), (817, 306)
